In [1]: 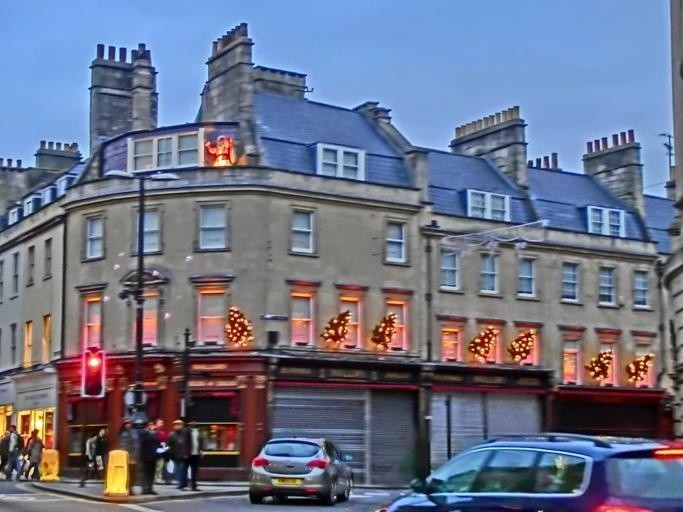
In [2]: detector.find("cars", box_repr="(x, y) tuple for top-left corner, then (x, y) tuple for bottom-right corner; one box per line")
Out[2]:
(249, 436), (353, 507)
(373, 430), (683, 512)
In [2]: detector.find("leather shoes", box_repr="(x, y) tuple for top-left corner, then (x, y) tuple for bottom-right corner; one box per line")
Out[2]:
(141, 483), (202, 495)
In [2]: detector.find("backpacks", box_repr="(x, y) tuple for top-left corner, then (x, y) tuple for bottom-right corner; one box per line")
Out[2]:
(1, 432), (24, 457)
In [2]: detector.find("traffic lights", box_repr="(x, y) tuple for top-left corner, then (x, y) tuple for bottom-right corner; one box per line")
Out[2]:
(80, 347), (107, 400)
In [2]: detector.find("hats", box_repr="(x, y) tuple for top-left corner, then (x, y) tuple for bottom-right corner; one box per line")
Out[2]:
(172, 419), (184, 425)
(122, 417), (131, 424)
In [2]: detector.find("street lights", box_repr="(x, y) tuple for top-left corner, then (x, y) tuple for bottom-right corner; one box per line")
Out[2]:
(104, 166), (182, 493)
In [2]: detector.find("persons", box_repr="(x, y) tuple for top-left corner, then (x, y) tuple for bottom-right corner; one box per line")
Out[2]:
(121, 417), (204, 488)
(0, 424), (44, 481)
(80, 428), (108, 487)
